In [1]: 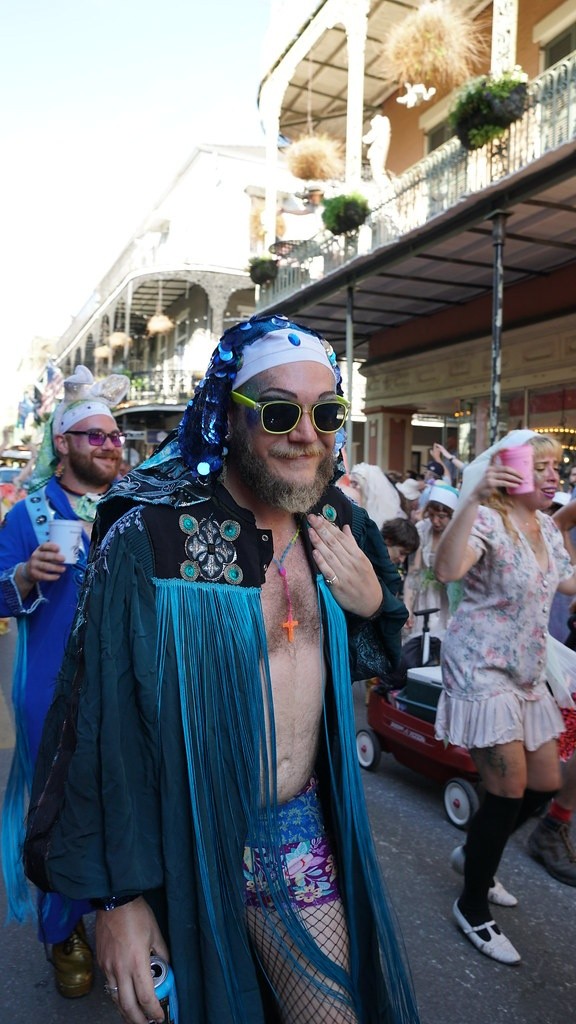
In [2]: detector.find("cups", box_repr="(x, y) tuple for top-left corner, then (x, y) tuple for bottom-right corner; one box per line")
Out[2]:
(49, 518), (83, 566)
(499, 444), (535, 494)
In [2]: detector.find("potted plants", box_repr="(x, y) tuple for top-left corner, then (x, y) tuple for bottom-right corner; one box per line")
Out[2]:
(245, 257), (280, 287)
(316, 194), (372, 238)
(447, 67), (530, 152)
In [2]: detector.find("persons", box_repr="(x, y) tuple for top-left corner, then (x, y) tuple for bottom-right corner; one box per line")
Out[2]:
(23, 314), (421, 1024)
(296, 180), (342, 276)
(347, 442), (470, 692)
(0, 363), (129, 998)
(431, 428), (576, 964)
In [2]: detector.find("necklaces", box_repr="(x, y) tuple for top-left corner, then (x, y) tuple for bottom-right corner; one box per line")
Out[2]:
(269, 518), (300, 642)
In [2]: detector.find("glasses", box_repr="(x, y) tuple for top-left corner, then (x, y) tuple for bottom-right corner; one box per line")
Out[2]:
(64, 430), (127, 446)
(232, 391), (351, 434)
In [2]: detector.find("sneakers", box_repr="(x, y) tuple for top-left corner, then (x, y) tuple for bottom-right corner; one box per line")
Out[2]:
(527, 821), (576, 887)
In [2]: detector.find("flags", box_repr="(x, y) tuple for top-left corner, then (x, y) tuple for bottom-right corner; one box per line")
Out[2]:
(42, 357), (64, 414)
(17, 392), (44, 432)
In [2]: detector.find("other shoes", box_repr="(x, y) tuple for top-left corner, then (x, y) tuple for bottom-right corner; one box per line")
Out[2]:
(453, 897), (522, 964)
(451, 846), (518, 906)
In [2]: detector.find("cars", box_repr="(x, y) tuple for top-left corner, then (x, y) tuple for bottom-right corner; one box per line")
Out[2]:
(0, 466), (26, 491)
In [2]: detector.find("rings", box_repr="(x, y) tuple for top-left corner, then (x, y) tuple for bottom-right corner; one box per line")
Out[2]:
(104, 984), (118, 996)
(326, 575), (337, 586)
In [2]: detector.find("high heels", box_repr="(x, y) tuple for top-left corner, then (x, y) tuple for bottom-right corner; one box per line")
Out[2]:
(54, 921), (93, 997)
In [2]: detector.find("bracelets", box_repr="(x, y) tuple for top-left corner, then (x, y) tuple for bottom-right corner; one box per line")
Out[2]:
(448, 454), (456, 462)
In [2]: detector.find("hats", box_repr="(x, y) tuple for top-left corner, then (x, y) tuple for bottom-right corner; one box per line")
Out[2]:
(395, 478), (420, 500)
(430, 480), (458, 510)
(421, 462), (444, 476)
